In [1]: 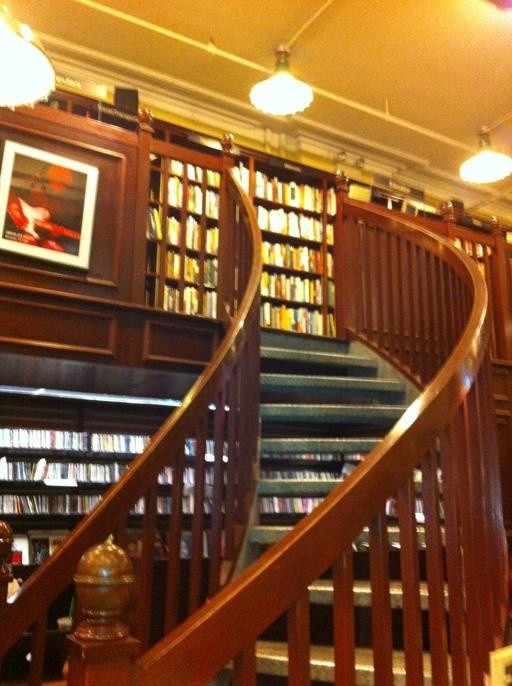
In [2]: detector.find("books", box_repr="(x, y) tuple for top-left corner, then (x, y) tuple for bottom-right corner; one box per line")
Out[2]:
(146, 151), (492, 338)
(1, 427), (446, 566)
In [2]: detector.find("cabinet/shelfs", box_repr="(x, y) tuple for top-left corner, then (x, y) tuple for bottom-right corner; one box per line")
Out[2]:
(0, 425), (446, 566)
(146, 128), (338, 340)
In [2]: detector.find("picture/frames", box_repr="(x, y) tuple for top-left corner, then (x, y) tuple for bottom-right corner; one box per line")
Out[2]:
(0, 139), (99, 270)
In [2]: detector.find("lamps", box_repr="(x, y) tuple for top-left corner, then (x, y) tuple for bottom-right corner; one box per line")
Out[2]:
(0, 0), (57, 112)
(459, 126), (512, 187)
(249, 43), (313, 118)
